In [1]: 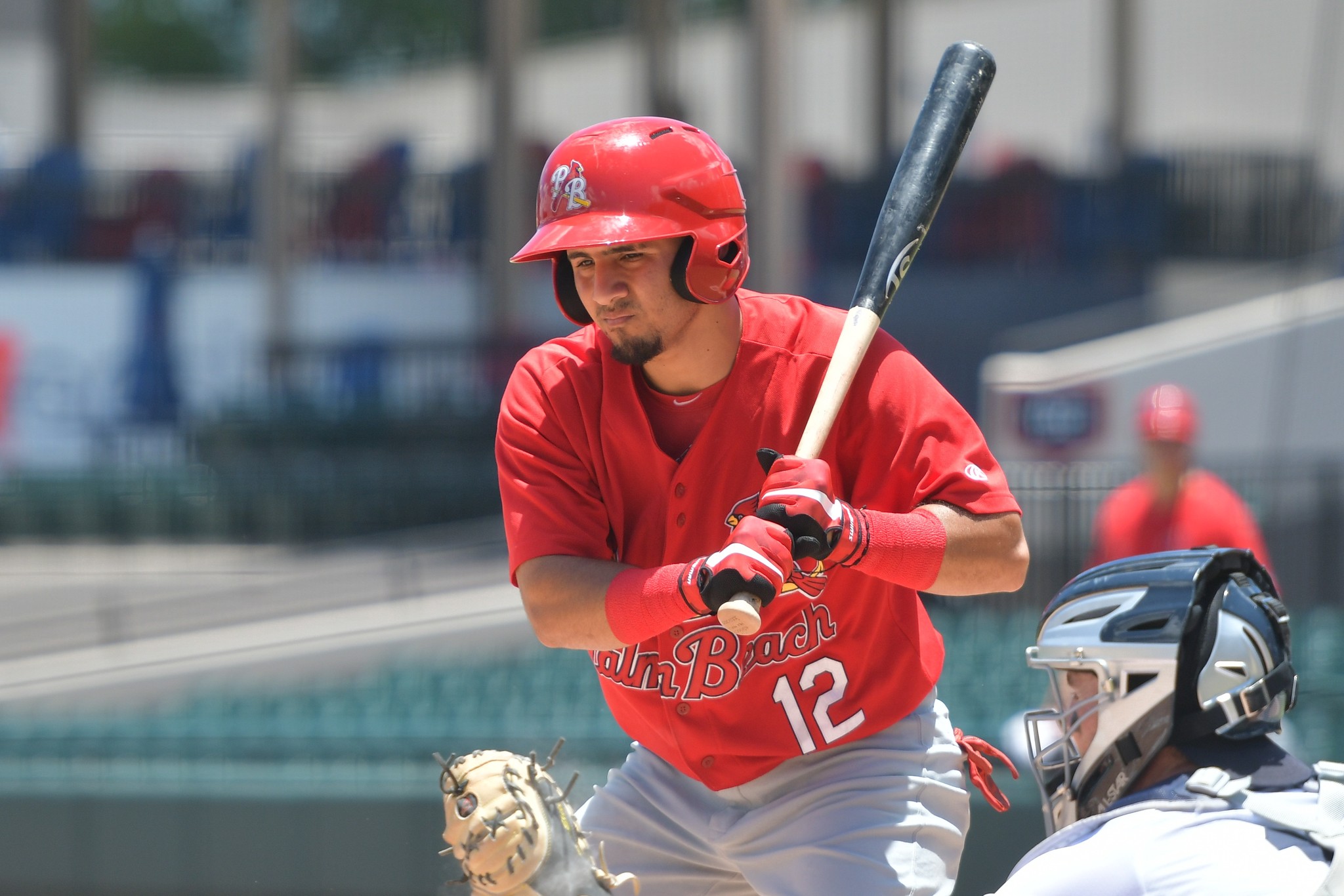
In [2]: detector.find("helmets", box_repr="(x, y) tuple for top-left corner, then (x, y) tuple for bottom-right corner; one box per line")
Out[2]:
(1141, 383), (1197, 445)
(1023, 545), (1300, 837)
(510, 117), (750, 326)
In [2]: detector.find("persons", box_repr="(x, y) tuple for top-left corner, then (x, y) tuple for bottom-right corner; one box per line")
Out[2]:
(1081, 383), (1279, 594)
(492, 115), (1032, 896)
(983, 541), (1344, 896)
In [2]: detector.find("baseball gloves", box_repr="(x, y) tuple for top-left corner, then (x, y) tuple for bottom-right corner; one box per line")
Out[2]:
(443, 749), (616, 896)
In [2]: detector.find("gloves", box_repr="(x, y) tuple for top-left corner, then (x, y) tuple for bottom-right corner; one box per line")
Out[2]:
(953, 727), (1019, 813)
(755, 448), (862, 564)
(677, 515), (795, 617)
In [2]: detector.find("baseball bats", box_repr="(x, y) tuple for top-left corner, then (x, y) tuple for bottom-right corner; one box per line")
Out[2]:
(713, 43), (998, 637)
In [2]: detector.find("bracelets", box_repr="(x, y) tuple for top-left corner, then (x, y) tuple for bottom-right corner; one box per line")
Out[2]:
(842, 504), (870, 566)
